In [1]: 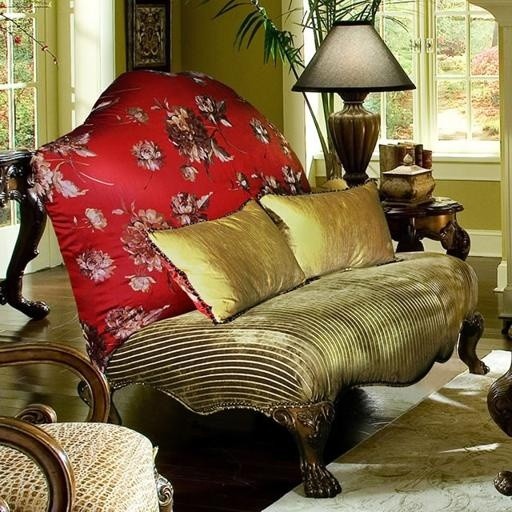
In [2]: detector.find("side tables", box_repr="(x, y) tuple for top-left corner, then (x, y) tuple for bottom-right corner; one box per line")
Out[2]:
(380, 196), (470, 261)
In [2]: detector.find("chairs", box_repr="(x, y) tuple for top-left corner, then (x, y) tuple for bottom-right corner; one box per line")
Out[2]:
(1, 344), (173, 512)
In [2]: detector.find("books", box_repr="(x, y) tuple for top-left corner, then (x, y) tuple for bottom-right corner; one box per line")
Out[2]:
(377, 142), (407, 191)
(421, 149), (433, 176)
(397, 141), (423, 168)
(387, 143), (415, 166)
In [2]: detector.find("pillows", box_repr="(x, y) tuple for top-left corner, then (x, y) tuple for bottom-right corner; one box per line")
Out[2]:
(143, 179), (401, 325)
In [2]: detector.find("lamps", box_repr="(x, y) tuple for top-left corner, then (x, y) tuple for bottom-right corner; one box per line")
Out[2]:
(291, 20), (416, 187)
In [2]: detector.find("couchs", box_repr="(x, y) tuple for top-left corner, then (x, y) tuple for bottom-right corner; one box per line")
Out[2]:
(31, 69), (490, 496)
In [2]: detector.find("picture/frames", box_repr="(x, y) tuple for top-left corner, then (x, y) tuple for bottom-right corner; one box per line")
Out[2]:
(125, 0), (171, 72)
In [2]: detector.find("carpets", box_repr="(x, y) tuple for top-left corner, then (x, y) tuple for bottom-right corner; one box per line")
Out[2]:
(260, 349), (512, 510)
